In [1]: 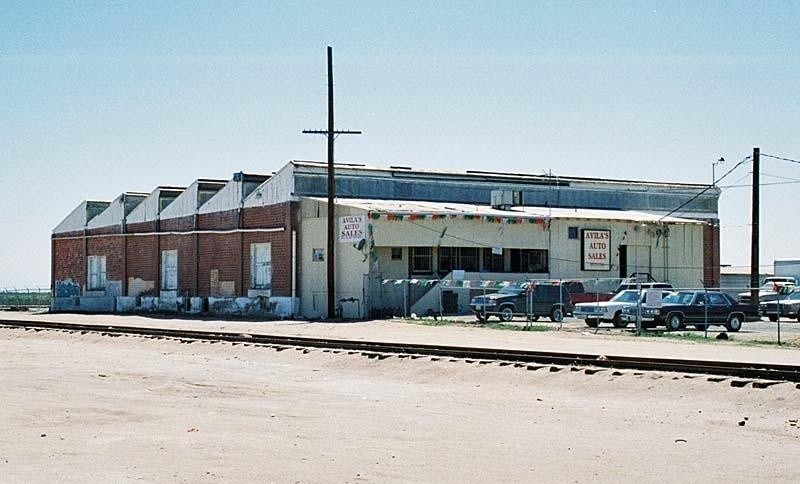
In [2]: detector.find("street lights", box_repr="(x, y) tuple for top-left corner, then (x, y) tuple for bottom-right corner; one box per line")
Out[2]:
(712, 157), (725, 188)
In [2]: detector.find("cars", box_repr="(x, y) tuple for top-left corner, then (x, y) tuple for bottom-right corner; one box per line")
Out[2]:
(469, 279), (745, 331)
(738, 277), (800, 322)
